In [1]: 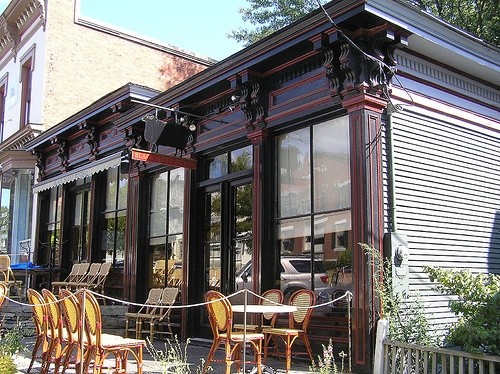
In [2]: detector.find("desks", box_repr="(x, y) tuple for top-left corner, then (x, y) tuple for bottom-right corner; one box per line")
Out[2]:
(231, 303), (297, 374)
(0, 261), (51, 301)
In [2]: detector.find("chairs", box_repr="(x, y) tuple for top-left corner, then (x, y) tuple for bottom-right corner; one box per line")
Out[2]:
(0, 254), (178, 374)
(201, 288), (317, 374)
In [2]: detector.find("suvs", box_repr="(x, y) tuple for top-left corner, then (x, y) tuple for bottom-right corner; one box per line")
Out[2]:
(209, 255), (329, 310)
